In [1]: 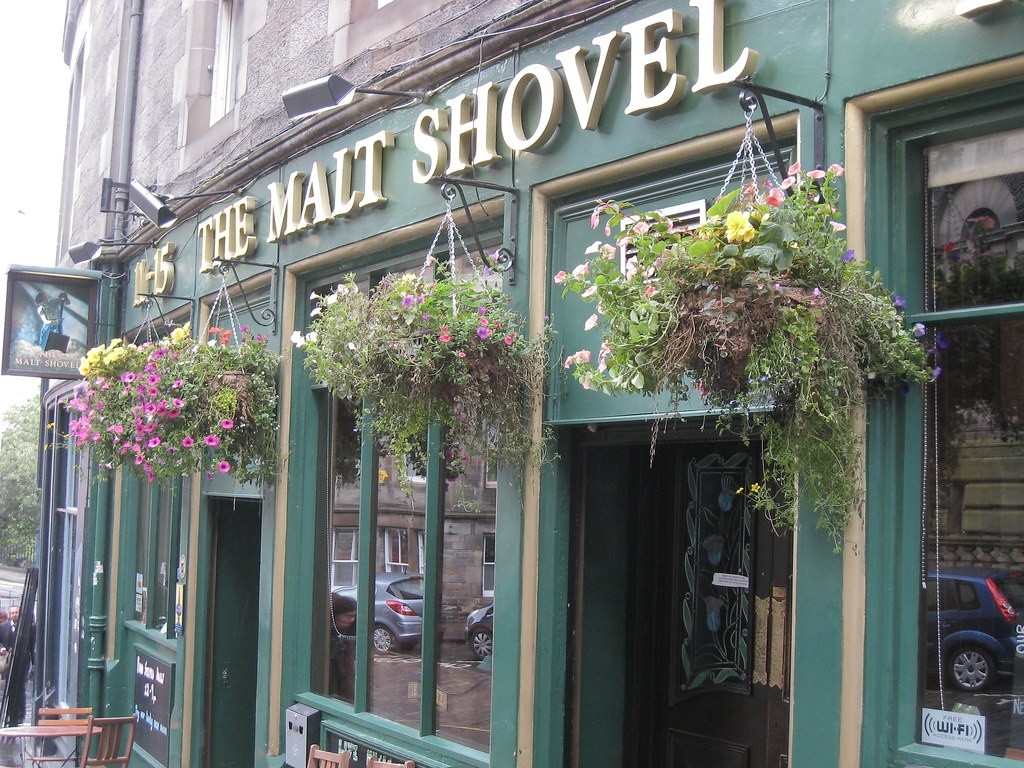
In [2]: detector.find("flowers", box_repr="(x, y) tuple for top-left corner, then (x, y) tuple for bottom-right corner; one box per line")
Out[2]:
(290, 250), (552, 516)
(43, 317), (283, 495)
(552, 161), (936, 555)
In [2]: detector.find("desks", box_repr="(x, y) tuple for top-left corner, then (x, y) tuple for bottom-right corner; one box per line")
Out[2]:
(0, 726), (101, 768)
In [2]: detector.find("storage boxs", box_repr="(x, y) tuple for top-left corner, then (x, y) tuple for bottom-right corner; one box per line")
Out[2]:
(286, 702), (322, 768)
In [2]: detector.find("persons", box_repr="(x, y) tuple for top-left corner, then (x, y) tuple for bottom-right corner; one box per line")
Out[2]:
(0, 605), (36, 724)
(328, 591), (357, 706)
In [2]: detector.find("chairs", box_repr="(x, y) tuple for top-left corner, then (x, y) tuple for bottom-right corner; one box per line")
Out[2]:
(26, 707), (92, 768)
(79, 712), (138, 768)
(307, 744), (349, 768)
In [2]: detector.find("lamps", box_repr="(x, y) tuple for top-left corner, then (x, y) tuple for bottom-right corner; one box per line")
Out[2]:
(128, 179), (244, 229)
(281, 76), (432, 122)
(67, 239), (156, 265)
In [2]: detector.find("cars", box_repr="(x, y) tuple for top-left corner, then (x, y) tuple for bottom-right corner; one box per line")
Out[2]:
(464, 599), (493, 660)
(924, 567), (1024, 693)
(375, 569), (423, 652)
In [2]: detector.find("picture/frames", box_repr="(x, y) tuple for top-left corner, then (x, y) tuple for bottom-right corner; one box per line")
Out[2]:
(1, 272), (98, 380)
